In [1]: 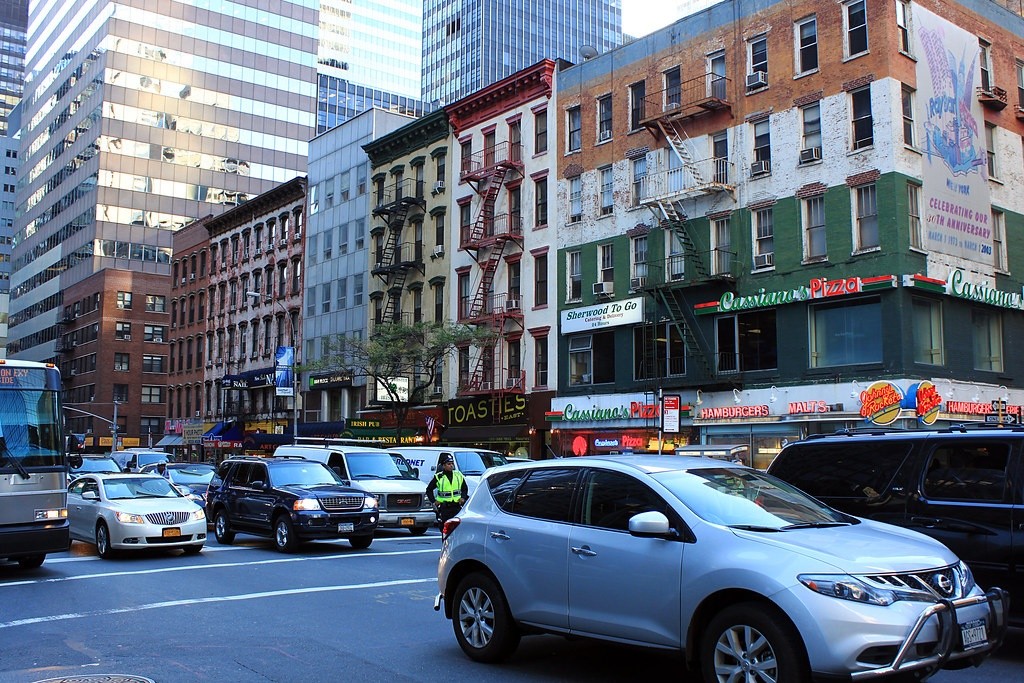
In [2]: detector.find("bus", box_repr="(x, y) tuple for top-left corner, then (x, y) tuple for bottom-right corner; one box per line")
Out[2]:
(124, 447), (187, 456)
(0, 358), (83, 570)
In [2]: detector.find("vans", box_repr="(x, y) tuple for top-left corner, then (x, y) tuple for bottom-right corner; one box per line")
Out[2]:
(111, 451), (177, 478)
(274, 438), (435, 535)
(386, 447), (508, 525)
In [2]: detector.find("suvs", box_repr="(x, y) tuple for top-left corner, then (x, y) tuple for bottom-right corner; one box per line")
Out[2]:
(206, 454), (379, 552)
(754, 425), (1024, 628)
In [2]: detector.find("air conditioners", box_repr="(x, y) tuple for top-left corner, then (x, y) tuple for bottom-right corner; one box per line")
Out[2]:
(482, 381), (492, 391)
(754, 253), (773, 267)
(294, 233), (301, 239)
(432, 181), (444, 188)
(114, 365), (121, 370)
(124, 335), (130, 339)
(268, 244), (275, 250)
(593, 282), (613, 294)
(665, 103), (681, 111)
(188, 274), (195, 280)
(601, 130), (610, 140)
(280, 238), (287, 245)
(799, 147), (820, 162)
(434, 386), (441, 394)
(507, 299), (520, 309)
(746, 71), (766, 88)
(630, 277), (645, 288)
(433, 245), (444, 254)
(506, 378), (520, 387)
(155, 338), (162, 342)
(196, 411), (201, 416)
(750, 161), (769, 175)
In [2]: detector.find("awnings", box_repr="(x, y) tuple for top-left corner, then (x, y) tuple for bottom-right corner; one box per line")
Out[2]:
(155, 434), (183, 445)
(441, 425), (525, 441)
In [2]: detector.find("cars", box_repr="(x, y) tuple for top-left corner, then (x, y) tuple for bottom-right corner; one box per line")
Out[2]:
(434, 451), (1010, 683)
(494, 457), (538, 467)
(67, 452), (124, 492)
(140, 463), (221, 511)
(66, 469), (207, 558)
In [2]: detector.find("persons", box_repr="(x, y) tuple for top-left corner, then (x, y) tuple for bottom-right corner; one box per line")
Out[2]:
(426, 453), (468, 534)
(149, 460), (169, 479)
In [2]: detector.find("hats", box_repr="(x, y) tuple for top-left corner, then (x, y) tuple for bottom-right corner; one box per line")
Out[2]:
(440, 454), (453, 465)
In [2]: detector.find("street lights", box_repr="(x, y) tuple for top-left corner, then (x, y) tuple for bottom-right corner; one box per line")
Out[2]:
(245, 291), (298, 444)
